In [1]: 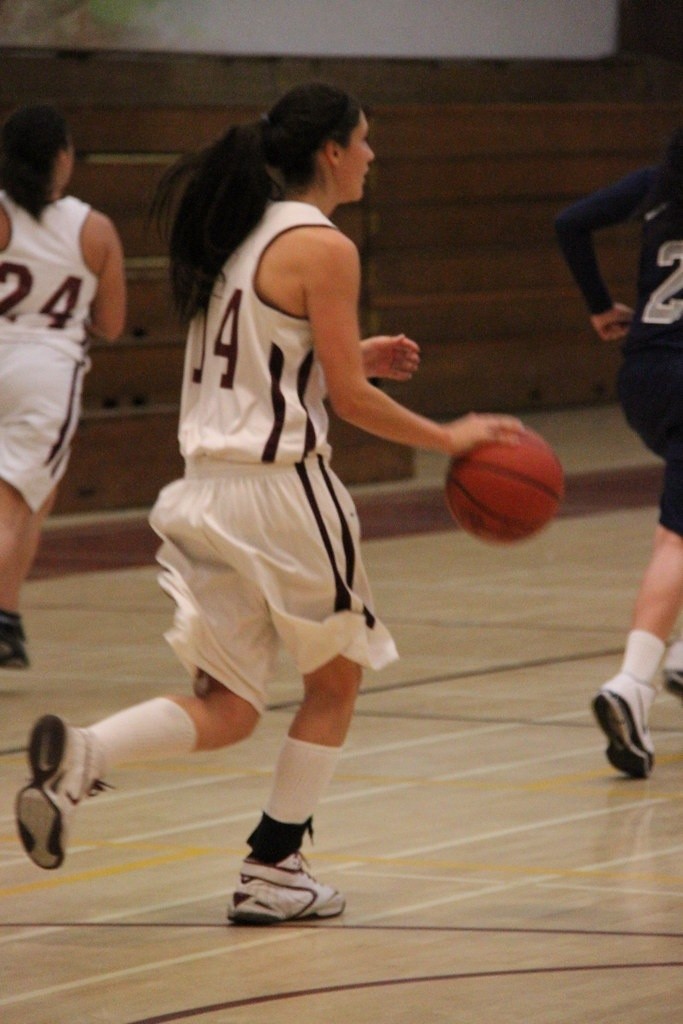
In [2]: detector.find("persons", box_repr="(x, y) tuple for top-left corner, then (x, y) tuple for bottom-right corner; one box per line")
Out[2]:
(552, 132), (682, 781)
(15, 82), (548, 925)
(0, 107), (125, 669)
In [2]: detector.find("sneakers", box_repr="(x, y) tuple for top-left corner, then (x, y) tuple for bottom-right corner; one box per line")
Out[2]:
(228, 850), (346, 923)
(592, 673), (656, 779)
(663, 641), (683, 699)
(15, 713), (115, 870)
(0, 609), (30, 667)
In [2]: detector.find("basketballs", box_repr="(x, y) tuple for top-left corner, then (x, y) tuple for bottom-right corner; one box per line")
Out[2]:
(440, 427), (566, 546)
